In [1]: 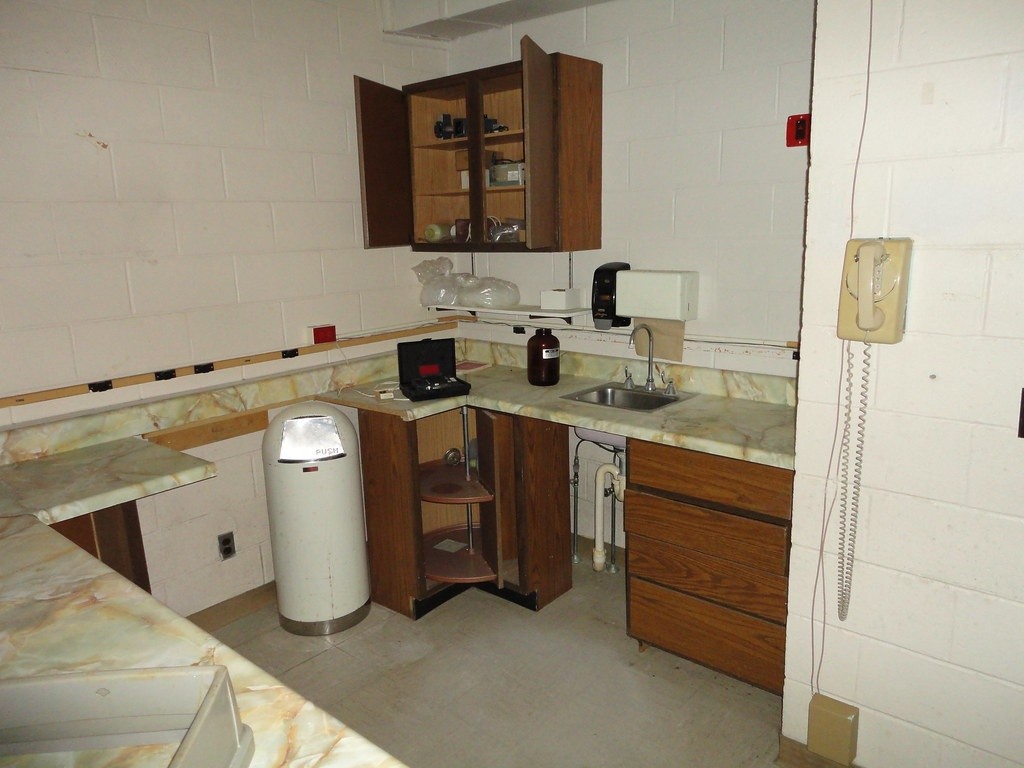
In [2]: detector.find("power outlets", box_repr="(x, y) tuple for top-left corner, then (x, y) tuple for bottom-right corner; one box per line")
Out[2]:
(218, 531), (236, 559)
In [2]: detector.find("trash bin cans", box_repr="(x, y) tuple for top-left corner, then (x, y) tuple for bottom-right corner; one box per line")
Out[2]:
(261, 401), (373, 635)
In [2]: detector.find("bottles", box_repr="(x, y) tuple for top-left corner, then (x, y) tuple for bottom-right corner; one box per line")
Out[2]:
(424, 224), (451, 242)
(527, 329), (560, 386)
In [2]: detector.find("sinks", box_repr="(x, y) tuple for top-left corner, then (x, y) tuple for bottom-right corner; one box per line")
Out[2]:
(559, 382), (701, 447)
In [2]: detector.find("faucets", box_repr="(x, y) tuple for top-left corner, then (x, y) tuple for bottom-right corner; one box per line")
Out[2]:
(628, 324), (657, 393)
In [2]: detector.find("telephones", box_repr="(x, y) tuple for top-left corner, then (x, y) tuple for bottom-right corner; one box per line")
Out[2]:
(836, 237), (915, 345)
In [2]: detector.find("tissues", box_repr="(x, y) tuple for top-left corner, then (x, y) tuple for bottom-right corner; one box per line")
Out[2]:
(616, 269), (698, 362)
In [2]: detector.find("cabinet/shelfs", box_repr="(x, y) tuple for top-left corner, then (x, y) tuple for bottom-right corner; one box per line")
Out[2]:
(352, 35), (604, 254)
(358, 407), (574, 621)
(623, 437), (794, 696)
(47, 501), (153, 600)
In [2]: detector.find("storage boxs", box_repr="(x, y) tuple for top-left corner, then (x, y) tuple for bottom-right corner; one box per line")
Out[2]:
(454, 148), (504, 189)
(540, 287), (579, 311)
